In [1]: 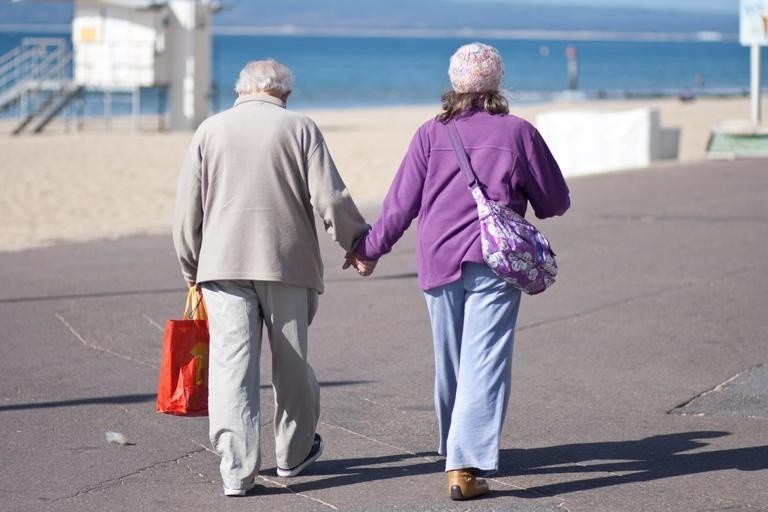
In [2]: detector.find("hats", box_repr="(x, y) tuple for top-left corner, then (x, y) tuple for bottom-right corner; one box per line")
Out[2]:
(447, 42), (505, 96)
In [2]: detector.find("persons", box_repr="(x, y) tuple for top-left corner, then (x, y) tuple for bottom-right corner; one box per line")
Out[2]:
(172, 59), (378, 496)
(339, 42), (571, 500)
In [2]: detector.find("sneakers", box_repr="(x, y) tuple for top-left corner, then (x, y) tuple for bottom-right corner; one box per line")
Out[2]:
(223, 487), (248, 497)
(276, 432), (325, 478)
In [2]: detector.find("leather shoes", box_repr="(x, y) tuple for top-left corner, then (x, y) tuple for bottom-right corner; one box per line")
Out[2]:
(448, 466), (495, 501)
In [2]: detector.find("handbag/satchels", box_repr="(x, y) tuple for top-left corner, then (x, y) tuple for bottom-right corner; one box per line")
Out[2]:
(156, 283), (211, 418)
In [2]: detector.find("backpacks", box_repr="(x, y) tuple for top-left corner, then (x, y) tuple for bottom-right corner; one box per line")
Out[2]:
(444, 115), (559, 296)
